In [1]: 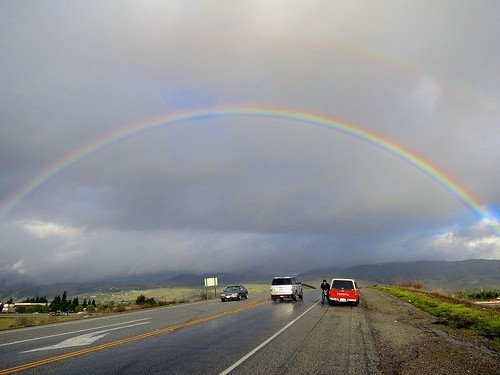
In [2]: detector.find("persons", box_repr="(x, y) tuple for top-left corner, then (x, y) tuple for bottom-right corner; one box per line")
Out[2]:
(320, 279), (331, 305)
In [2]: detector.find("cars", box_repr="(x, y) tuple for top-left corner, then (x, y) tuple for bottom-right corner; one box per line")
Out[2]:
(219, 284), (248, 302)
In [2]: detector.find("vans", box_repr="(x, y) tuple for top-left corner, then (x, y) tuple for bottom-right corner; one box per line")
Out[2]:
(270, 276), (304, 302)
(328, 278), (360, 307)
